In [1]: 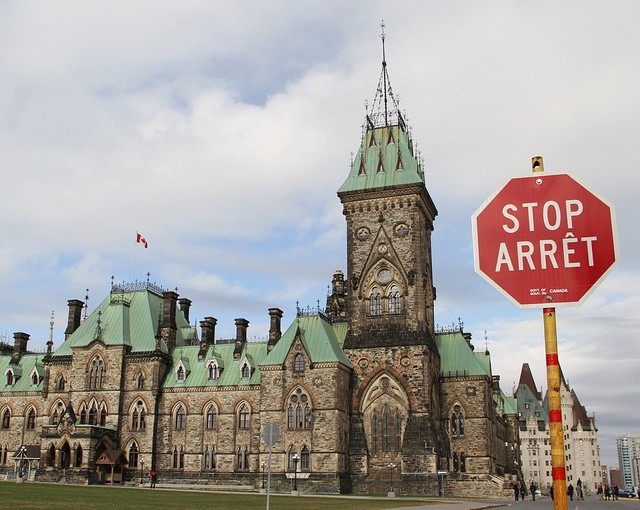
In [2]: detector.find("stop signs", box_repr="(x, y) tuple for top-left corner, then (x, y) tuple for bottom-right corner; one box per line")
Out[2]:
(470, 170), (619, 309)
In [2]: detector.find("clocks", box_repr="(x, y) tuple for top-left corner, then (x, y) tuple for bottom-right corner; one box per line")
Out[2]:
(377, 243), (388, 253)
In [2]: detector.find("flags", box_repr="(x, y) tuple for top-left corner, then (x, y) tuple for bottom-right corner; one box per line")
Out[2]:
(136, 233), (148, 248)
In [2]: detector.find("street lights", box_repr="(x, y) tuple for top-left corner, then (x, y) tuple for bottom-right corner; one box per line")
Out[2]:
(387, 461), (396, 492)
(260, 461), (266, 490)
(290, 453), (301, 491)
(18, 445), (27, 483)
(139, 457), (145, 485)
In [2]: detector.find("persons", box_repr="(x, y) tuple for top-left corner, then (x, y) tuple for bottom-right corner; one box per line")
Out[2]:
(549, 482), (554, 501)
(612, 484), (619, 500)
(520, 485), (526, 500)
(605, 485), (611, 501)
(513, 485), (519, 501)
(567, 484), (573, 501)
(596, 484), (603, 501)
(529, 480), (537, 501)
(575, 478), (584, 501)
(150, 469), (158, 488)
